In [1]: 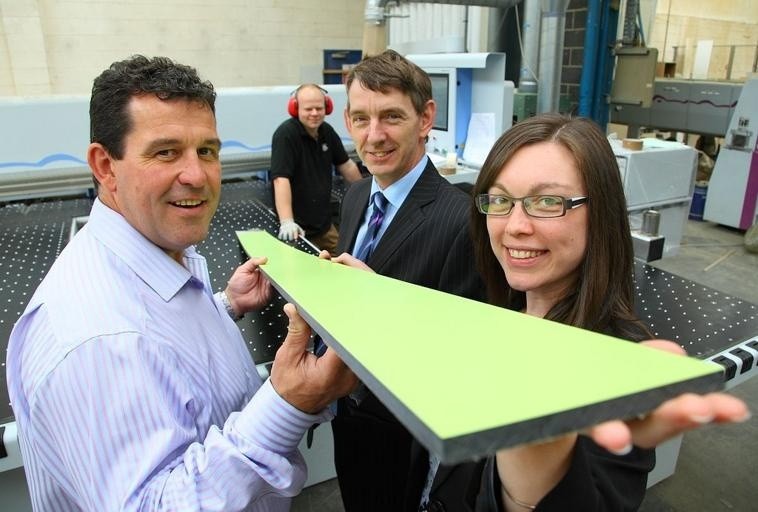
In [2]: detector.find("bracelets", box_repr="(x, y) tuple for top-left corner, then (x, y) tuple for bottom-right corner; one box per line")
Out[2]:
(501, 481), (536, 510)
(220, 289), (235, 319)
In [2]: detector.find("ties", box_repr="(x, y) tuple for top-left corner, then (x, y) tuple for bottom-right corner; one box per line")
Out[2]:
(316, 194), (388, 356)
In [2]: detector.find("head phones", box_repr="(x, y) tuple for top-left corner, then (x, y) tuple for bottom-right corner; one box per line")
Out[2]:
(287, 83), (333, 117)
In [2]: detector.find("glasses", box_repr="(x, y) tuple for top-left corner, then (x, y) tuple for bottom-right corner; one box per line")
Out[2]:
(475, 195), (589, 219)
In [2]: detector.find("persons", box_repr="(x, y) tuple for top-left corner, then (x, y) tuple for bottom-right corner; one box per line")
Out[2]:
(3, 54), (358, 512)
(266, 83), (363, 243)
(317, 47), (482, 511)
(405, 110), (754, 512)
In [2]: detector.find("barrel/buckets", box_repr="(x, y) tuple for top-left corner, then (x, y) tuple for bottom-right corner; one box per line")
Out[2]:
(687, 184), (708, 222)
